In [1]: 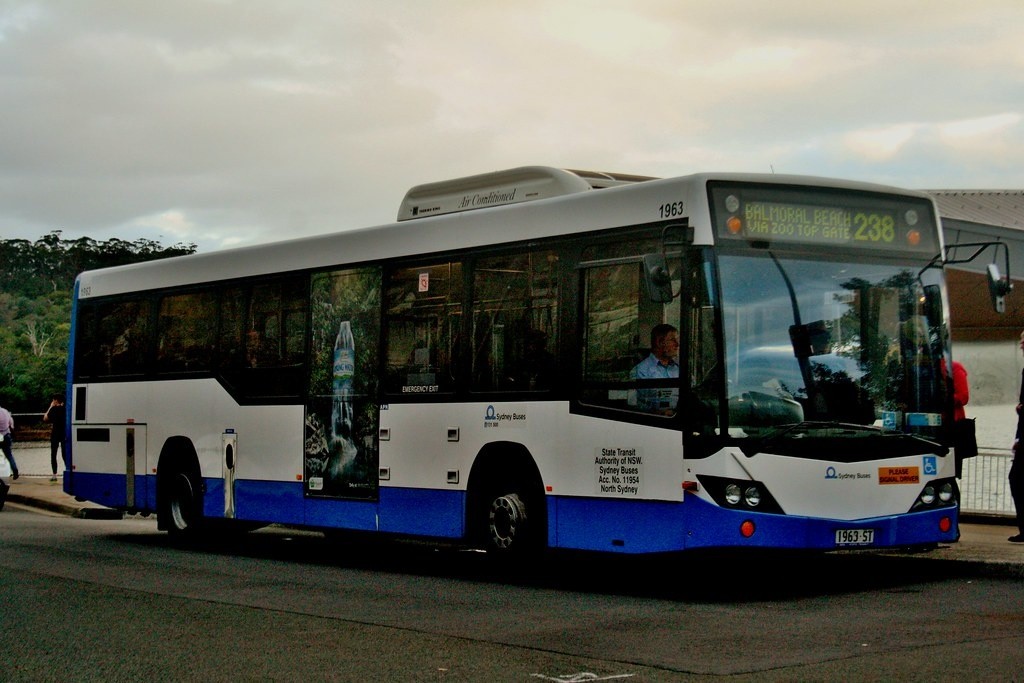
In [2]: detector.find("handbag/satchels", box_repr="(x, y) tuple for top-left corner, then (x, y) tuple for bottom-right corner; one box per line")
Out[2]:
(955, 417), (978, 459)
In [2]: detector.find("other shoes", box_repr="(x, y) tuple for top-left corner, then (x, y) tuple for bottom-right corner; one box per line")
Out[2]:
(1008, 534), (1024, 542)
(13, 473), (19, 480)
(50, 478), (57, 481)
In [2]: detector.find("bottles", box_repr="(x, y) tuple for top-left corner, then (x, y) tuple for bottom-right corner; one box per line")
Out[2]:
(331, 321), (355, 399)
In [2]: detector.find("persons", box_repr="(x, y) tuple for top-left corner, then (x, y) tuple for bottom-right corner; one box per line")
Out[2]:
(1007, 330), (1024, 543)
(939, 357), (969, 543)
(627, 323), (696, 416)
(43, 393), (66, 483)
(0, 406), (19, 481)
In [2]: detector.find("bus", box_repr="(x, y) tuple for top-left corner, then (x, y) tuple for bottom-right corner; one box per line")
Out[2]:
(60, 166), (1014, 575)
